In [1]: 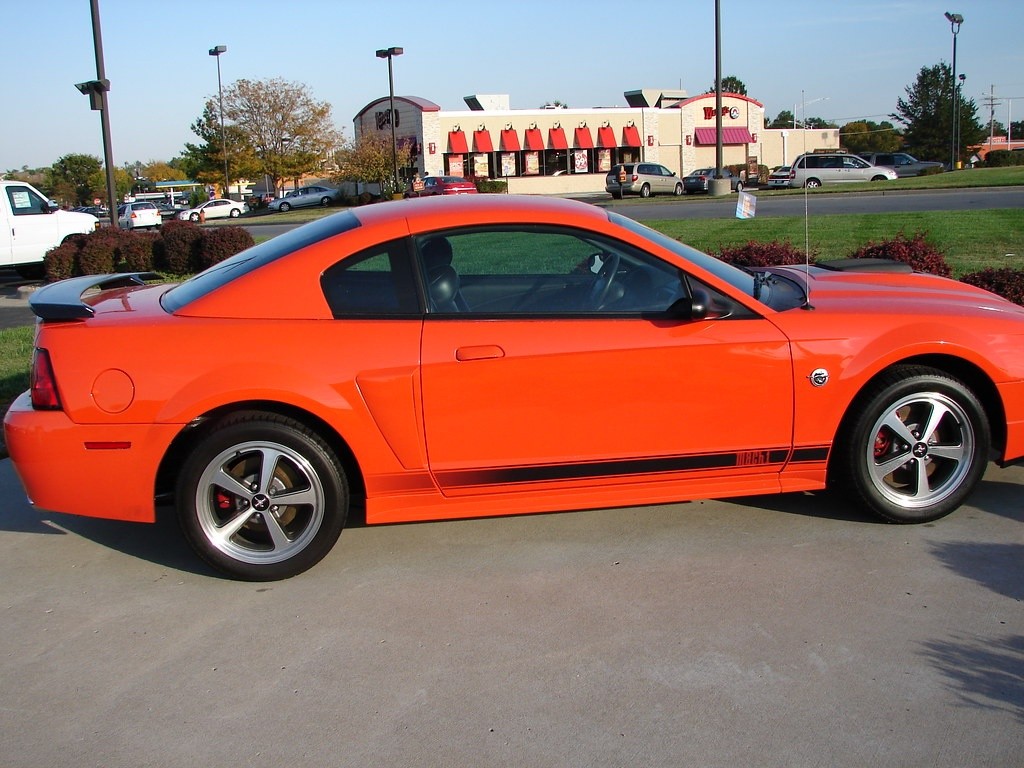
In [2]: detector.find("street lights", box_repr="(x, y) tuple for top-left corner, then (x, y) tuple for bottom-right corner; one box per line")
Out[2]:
(956, 73), (966, 172)
(209, 44), (231, 201)
(944, 12), (964, 171)
(376, 47), (404, 201)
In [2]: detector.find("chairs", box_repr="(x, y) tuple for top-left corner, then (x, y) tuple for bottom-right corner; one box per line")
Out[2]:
(422, 237), (453, 271)
(427, 264), (472, 312)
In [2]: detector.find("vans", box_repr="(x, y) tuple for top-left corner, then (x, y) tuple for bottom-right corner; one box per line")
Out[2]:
(788, 149), (898, 190)
(604, 163), (684, 197)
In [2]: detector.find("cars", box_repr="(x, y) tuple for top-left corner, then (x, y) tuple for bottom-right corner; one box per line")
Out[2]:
(770, 164), (796, 189)
(267, 185), (336, 210)
(854, 152), (943, 179)
(683, 169), (744, 193)
(181, 198), (250, 222)
(0, 194), (1023, 569)
(402, 175), (478, 201)
(0, 168), (184, 272)
